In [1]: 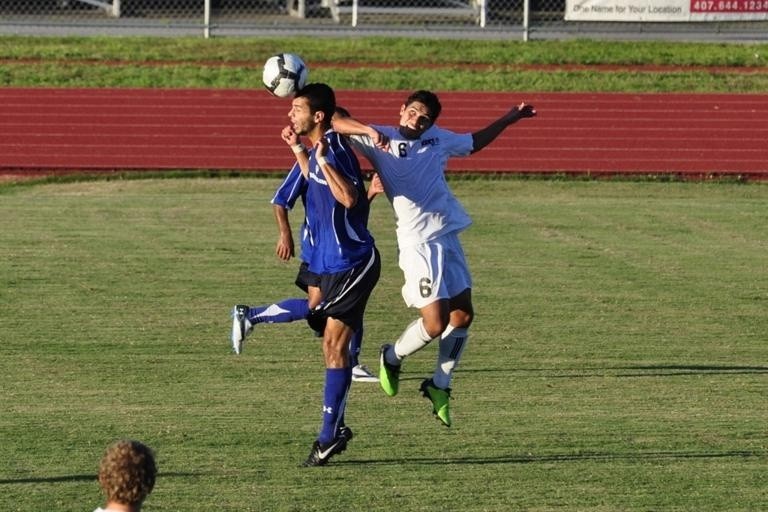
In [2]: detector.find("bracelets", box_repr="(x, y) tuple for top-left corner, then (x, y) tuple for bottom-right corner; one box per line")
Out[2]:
(289, 143), (305, 155)
(318, 157), (330, 166)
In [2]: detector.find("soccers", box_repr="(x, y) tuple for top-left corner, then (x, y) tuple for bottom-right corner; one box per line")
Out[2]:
(262, 53), (306, 98)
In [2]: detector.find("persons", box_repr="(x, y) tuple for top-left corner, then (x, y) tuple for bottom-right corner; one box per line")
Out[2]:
(94, 439), (159, 512)
(280, 83), (380, 466)
(332, 89), (537, 428)
(233, 109), (384, 383)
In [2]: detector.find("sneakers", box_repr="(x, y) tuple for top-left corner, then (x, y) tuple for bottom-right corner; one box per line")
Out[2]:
(350, 366), (376, 382)
(300, 436), (345, 467)
(418, 378), (452, 427)
(232, 304), (251, 355)
(377, 344), (400, 398)
(338, 427), (352, 440)
